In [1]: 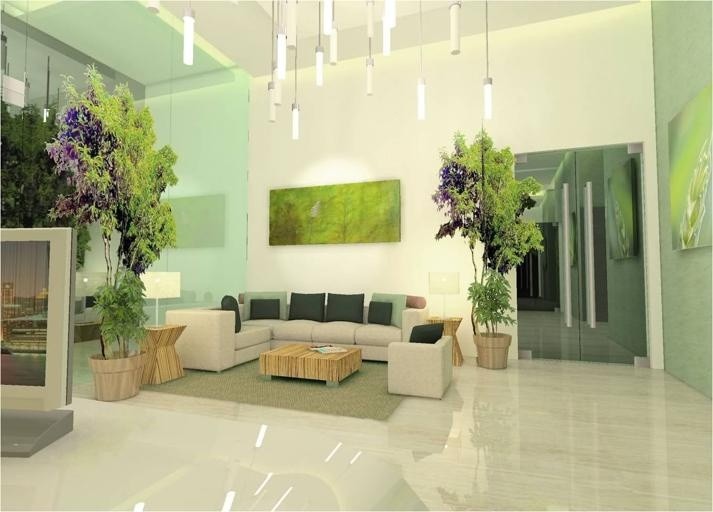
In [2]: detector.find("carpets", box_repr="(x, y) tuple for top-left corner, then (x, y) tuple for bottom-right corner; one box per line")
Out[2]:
(139, 368), (405, 420)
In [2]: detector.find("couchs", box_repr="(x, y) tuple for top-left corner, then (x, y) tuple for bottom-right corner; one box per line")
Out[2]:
(166, 306), (271, 374)
(237, 301), (430, 363)
(387, 334), (454, 401)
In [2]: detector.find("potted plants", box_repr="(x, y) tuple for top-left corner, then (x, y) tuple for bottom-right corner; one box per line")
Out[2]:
(46, 64), (180, 403)
(433, 128), (546, 370)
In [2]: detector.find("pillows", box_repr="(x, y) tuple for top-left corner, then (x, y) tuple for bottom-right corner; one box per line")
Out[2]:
(406, 295), (426, 309)
(325, 293), (364, 323)
(372, 293), (406, 329)
(242, 291), (287, 321)
(219, 295), (242, 333)
(368, 301), (392, 325)
(409, 322), (444, 344)
(248, 298), (281, 320)
(287, 291), (325, 323)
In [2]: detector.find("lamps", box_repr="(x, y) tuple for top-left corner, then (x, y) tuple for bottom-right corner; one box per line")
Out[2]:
(277, 0), (287, 80)
(274, 0), (283, 107)
(448, 0), (462, 56)
(329, 1), (338, 65)
(182, 2), (196, 67)
(417, 1), (426, 121)
(267, 1), (276, 124)
(292, 0), (300, 141)
(484, 0), (493, 121)
(366, 1), (375, 97)
(315, 1), (325, 88)
